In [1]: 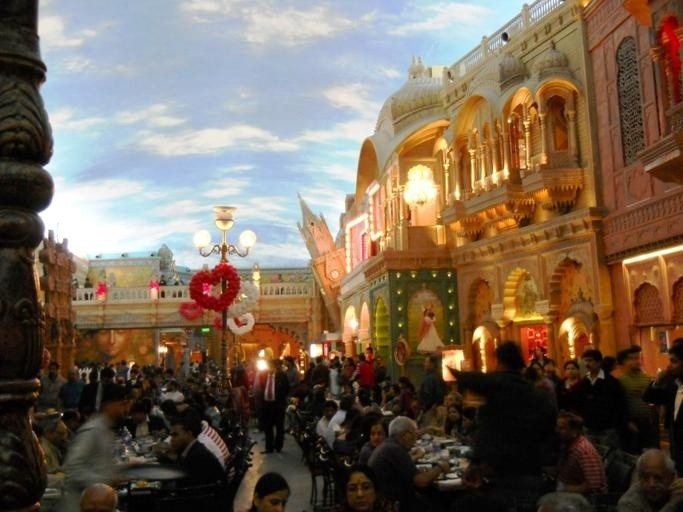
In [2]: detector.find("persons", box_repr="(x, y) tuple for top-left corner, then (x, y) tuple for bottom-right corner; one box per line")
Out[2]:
(157, 274), (166, 298)
(33, 340), (682, 511)
(427, 304), (434, 319)
(276, 273), (286, 294)
(71, 278), (80, 301)
(418, 304), (444, 353)
(83, 278), (95, 298)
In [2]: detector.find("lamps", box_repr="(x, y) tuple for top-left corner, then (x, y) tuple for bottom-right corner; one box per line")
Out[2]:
(403, 162), (437, 206)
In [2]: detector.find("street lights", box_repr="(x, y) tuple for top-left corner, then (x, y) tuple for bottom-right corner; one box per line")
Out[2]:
(190, 202), (255, 391)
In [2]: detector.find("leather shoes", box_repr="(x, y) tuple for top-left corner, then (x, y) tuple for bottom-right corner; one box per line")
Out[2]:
(260, 449), (273, 453)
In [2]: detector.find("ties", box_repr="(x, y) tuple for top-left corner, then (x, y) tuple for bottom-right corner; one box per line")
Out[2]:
(268, 375), (272, 400)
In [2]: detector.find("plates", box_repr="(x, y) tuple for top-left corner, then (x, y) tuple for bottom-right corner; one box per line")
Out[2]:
(415, 436), (473, 478)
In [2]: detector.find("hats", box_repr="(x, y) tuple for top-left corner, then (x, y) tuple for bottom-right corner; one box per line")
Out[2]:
(103, 383), (130, 402)
